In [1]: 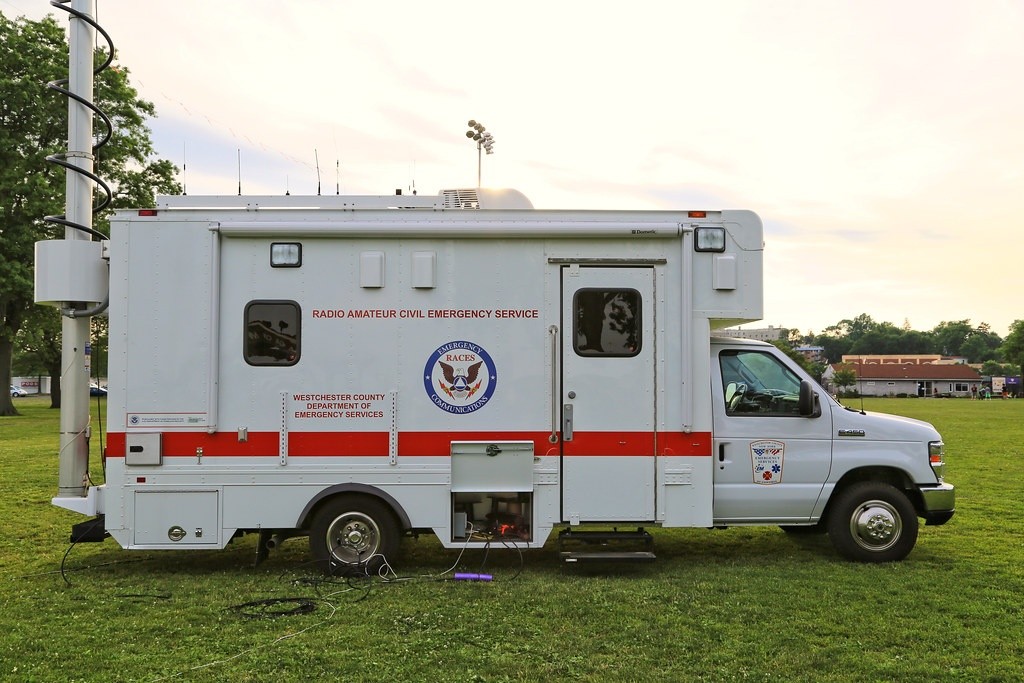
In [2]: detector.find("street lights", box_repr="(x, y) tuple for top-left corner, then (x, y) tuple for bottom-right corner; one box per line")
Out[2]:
(463, 116), (497, 186)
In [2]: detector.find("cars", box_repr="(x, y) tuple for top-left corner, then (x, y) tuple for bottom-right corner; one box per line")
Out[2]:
(90, 383), (107, 397)
(10, 385), (28, 397)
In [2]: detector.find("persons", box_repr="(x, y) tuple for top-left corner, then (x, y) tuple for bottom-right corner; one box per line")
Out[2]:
(979, 387), (992, 400)
(933, 388), (938, 397)
(970, 384), (977, 400)
(1002, 383), (1008, 400)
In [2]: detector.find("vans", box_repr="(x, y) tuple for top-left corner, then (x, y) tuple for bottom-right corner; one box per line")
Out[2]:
(31, 184), (955, 581)
